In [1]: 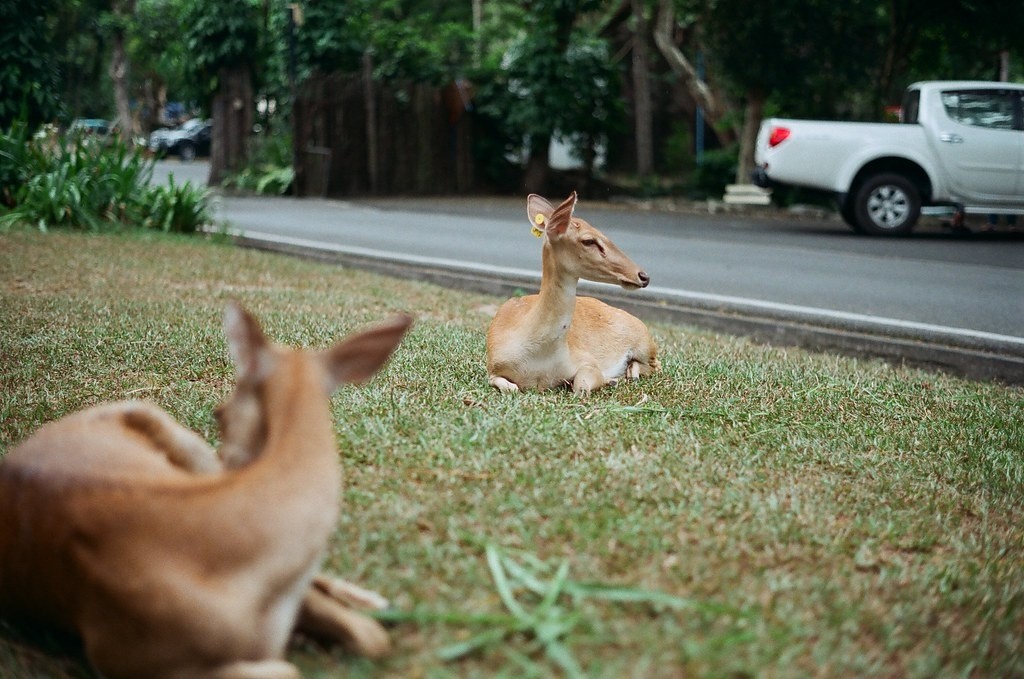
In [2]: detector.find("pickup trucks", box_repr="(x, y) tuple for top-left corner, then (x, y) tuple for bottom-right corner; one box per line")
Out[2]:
(755, 81), (1024, 239)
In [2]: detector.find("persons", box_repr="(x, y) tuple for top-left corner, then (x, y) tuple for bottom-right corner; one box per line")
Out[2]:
(131, 97), (158, 159)
(166, 99), (197, 131)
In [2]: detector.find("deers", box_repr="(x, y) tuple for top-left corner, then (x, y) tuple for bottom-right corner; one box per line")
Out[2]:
(0, 304), (415, 679)
(485, 191), (659, 398)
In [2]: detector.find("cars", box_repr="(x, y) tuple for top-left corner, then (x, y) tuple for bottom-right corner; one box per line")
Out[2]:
(150, 116), (213, 162)
(70, 118), (116, 148)
